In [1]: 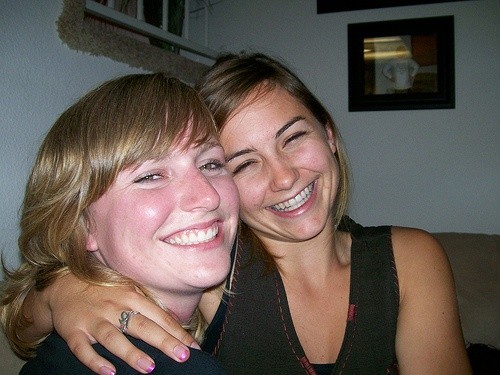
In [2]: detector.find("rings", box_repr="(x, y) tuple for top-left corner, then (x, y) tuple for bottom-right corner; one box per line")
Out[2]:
(119, 310), (140, 336)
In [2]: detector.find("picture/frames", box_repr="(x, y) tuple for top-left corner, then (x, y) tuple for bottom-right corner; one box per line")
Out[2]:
(347, 15), (455, 112)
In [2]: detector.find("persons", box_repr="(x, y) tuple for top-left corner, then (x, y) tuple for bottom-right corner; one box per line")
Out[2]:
(383, 46), (418, 93)
(0, 72), (240, 375)
(8, 51), (471, 375)
